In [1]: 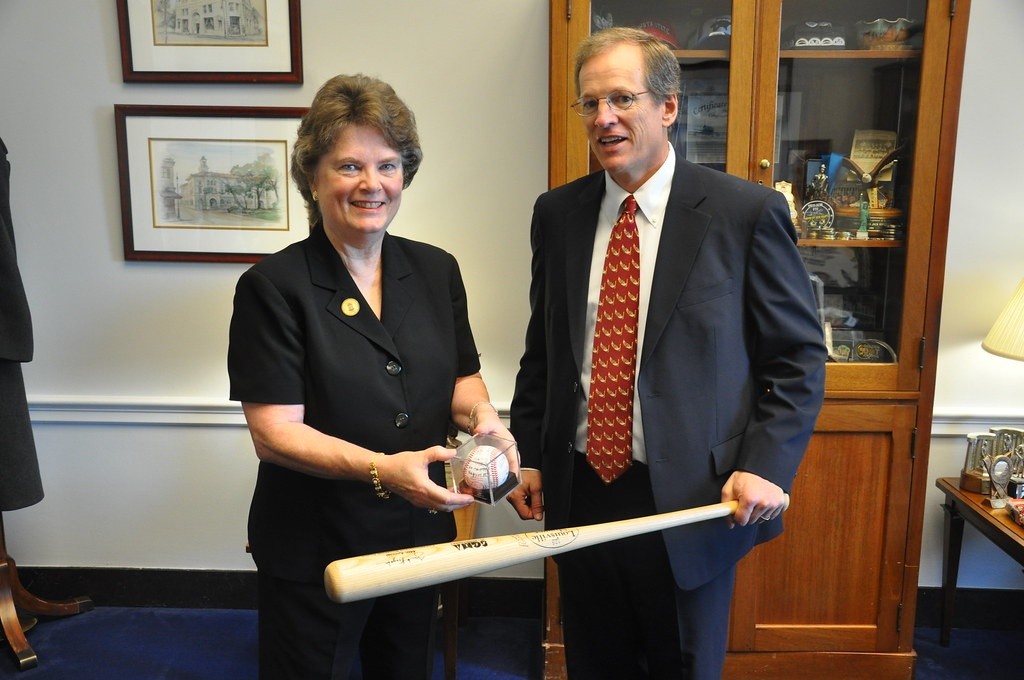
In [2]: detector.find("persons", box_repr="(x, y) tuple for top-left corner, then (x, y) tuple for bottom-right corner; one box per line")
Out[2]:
(225, 75), (520, 679)
(505, 27), (829, 679)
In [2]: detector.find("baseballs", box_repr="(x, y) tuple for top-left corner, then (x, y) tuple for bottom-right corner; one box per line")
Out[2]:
(462, 445), (509, 490)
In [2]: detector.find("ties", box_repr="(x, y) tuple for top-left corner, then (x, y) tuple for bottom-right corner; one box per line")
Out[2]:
(584, 196), (639, 486)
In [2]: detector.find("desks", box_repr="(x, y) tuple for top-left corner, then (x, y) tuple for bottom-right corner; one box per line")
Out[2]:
(244, 437), (479, 680)
(935, 477), (1024, 648)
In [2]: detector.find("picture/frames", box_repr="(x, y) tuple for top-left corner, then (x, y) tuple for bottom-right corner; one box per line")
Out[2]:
(116, 0), (305, 85)
(677, 83), (811, 175)
(114, 103), (317, 264)
(779, 139), (830, 200)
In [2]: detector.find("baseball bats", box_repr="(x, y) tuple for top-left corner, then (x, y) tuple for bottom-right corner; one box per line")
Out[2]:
(324, 498), (742, 608)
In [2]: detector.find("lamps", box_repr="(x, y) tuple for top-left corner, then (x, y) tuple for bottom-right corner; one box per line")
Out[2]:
(981, 278), (1024, 362)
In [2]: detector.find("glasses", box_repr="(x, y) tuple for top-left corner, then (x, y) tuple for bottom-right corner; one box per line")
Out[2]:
(571, 89), (651, 117)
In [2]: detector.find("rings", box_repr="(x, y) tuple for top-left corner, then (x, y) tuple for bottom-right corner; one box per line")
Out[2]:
(427, 508), (438, 514)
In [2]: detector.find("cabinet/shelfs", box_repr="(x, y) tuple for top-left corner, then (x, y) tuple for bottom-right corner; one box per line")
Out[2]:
(549, 0), (970, 680)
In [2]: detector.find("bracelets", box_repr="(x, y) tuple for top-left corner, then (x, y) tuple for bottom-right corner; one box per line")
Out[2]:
(369, 450), (392, 501)
